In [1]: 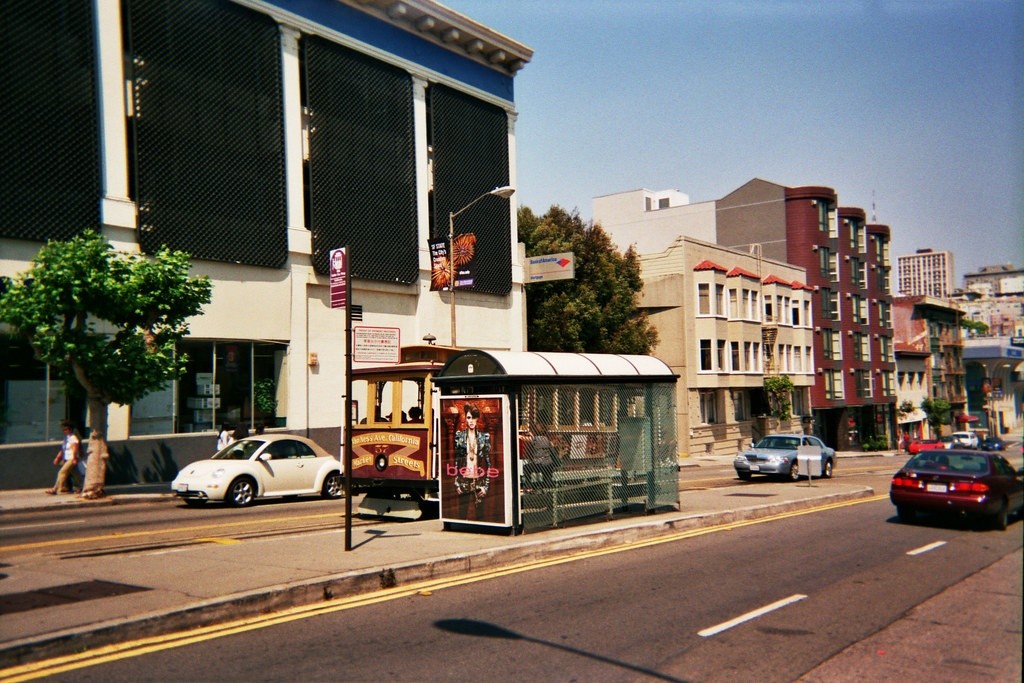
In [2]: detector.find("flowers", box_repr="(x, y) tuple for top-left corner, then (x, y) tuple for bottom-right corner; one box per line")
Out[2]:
(902, 400), (914, 405)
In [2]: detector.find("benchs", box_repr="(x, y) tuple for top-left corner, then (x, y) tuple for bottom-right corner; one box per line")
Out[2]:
(521, 467), (616, 528)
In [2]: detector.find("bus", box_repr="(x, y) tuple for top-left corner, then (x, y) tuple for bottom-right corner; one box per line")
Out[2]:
(340, 333), (637, 519)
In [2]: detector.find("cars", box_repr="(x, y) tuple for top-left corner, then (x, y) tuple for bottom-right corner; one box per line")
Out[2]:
(170, 434), (341, 509)
(907, 431), (1006, 454)
(733, 434), (837, 483)
(889, 447), (1024, 532)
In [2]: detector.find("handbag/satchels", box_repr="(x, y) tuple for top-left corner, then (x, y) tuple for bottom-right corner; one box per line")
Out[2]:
(549, 442), (561, 467)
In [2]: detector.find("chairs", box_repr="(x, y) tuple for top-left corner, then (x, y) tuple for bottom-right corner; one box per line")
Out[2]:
(962, 462), (985, 473)
(775, 439), (787, 446)
(937, 456), (958, 472)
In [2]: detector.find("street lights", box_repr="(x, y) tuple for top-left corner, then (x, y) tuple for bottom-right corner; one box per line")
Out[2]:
(450, 186), (517, 348)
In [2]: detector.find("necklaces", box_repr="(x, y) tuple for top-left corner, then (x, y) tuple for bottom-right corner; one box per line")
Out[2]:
(467, 434), (477, 461)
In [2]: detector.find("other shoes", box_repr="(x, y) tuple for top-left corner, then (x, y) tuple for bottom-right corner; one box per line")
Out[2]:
(61, 488), (71, 492)
(45, 489), (57, 495)
(74, 489), (82, 494)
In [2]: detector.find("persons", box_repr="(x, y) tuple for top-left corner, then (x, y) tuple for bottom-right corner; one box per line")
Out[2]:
(217, 422), (249, 452)
(454, 406), (491, 522)
(360, 405), (424, 424)
(255, 424), (265, 435)
(45, 420), (87, 495)
(519, 424), (559, 489)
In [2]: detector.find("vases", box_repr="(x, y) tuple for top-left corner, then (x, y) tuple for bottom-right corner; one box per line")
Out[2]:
(903, 404), (911, 407)
